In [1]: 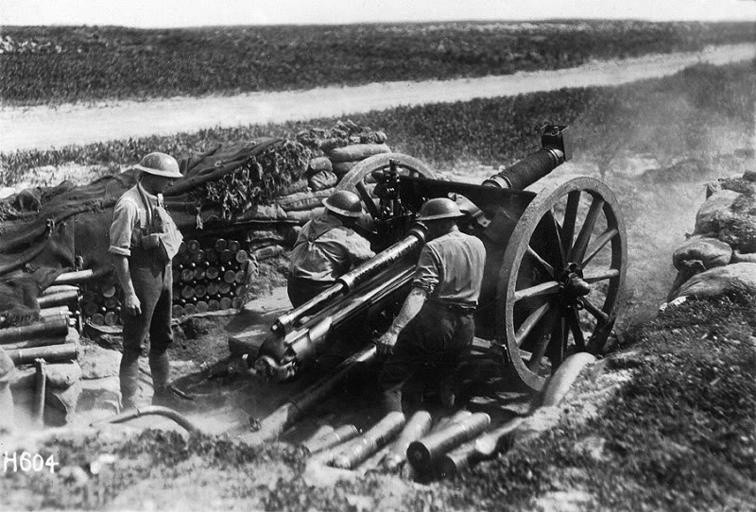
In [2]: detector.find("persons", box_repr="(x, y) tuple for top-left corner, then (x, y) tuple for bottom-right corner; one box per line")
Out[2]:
(105, 148), (196, 416)
(375, 195), (490, 418)
(285, 189), (376, 341)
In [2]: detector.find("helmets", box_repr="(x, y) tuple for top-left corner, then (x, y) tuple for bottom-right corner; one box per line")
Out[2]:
(133, 152), (185, 179)
(321, 190), (365, 219)
(413, 196), (465, 221)
